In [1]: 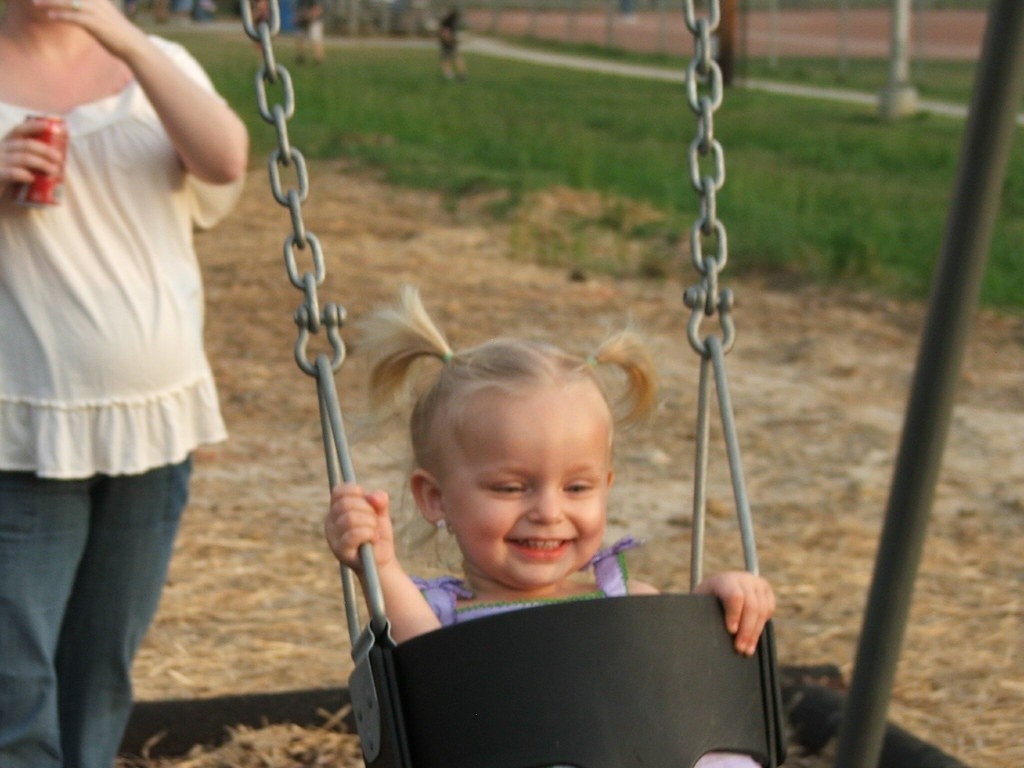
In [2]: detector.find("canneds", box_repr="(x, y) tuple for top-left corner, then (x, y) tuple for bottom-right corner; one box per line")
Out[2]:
(12, 115), (70, 205)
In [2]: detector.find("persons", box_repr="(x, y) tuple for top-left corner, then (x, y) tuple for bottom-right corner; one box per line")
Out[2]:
(125, 0), (216, 22)
(437, 8), (470, 81)
(709, 0), (740, 87)
(289, 0), (325, 64)
(2, 1), (251, 767)
(251, 0), (270, 64)
(326, 285), (776, 658)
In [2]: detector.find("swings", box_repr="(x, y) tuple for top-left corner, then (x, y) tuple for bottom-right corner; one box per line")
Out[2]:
(234, 1), (793, 767)
